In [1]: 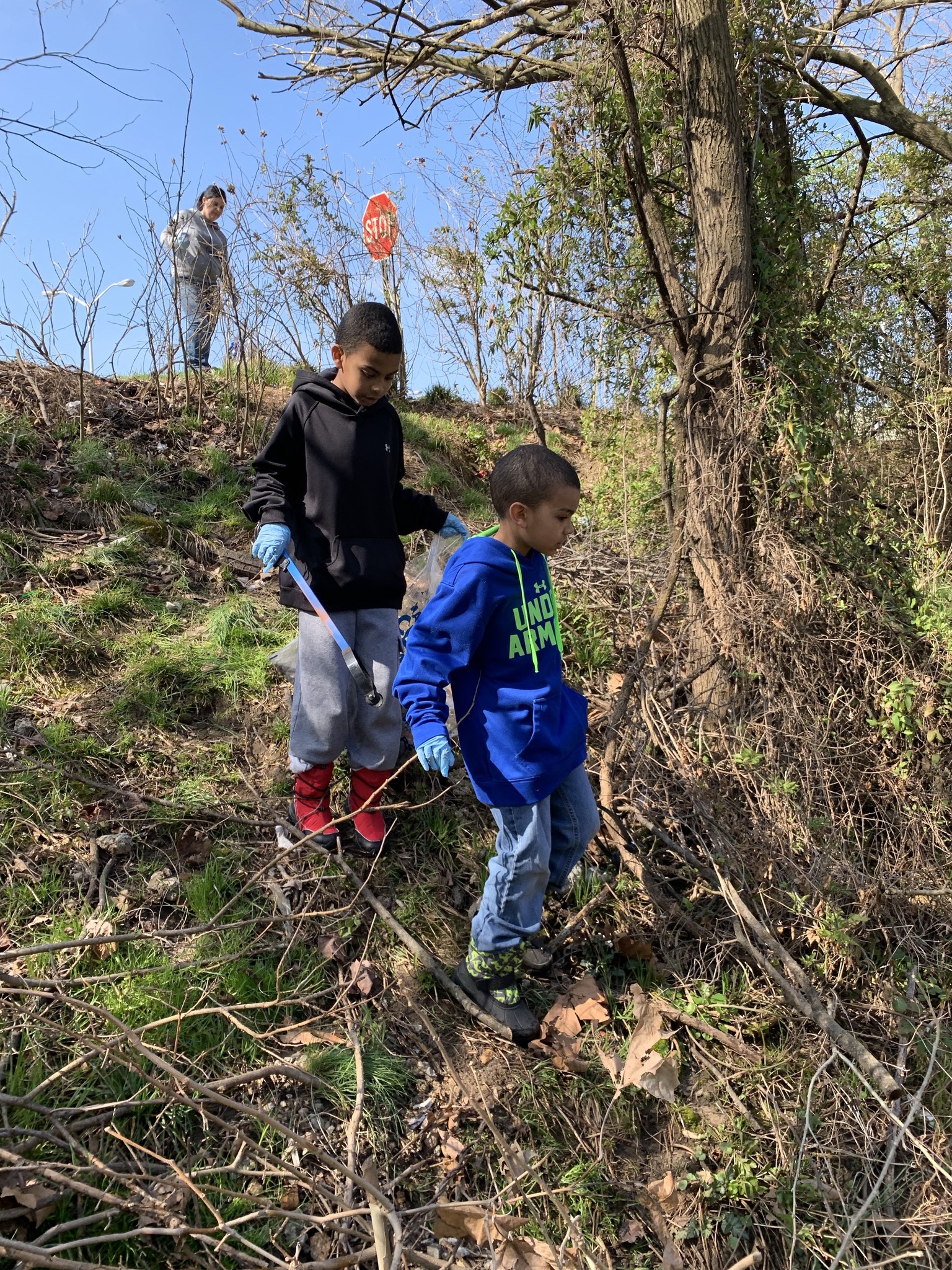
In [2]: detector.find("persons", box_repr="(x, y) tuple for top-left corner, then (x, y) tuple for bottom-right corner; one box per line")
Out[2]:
(242, 302), (468, 853)
(392, 443), (601, 1048)
(159, 185), (241, 369)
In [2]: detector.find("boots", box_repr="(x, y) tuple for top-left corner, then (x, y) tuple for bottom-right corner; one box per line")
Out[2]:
(344, 771), (393, 850)
(291, 762), (341, 844)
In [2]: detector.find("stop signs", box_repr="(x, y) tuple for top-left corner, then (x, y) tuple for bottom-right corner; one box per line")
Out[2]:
(361, 192), (399, 262)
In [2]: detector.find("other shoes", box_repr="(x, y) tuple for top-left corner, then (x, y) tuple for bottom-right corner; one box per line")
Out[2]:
(453, 964), (541, 1038)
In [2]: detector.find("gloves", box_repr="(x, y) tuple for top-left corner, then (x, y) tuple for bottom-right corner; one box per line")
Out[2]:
(416, 734), (454, 777)
(438, 515), (467, 538)
(252, 521), (291, 573)
(174, 231), (190, 250)
